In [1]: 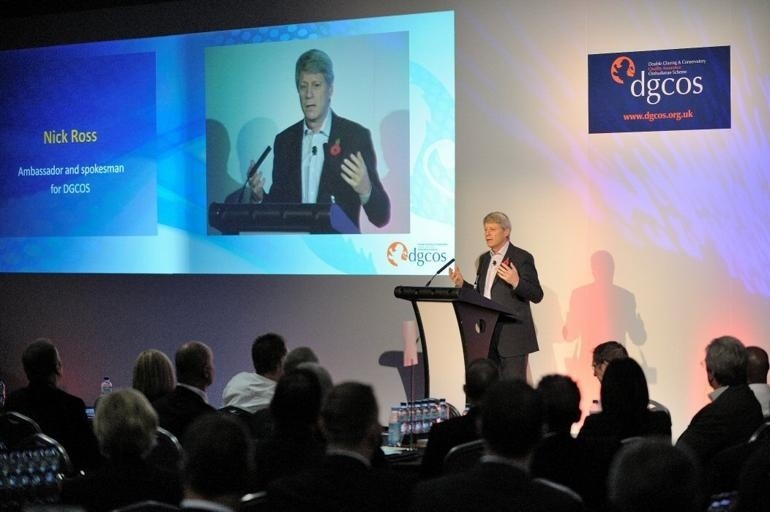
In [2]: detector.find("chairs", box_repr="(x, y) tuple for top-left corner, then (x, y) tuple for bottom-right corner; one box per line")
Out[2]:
(1, 411), (186, 463)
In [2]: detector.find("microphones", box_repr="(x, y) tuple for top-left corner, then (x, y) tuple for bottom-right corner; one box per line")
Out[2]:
(238, 146), (271, 204)
(426, 258), (455, 287)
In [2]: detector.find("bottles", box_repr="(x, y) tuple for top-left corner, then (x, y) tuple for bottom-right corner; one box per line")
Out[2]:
(388, 406), (402, 446)
(102, 376), (113, 394)
(400, 398), (450, 433)
(0, 444), (66, 488)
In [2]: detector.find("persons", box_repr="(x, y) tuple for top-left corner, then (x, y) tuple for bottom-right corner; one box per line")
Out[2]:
(577, 336), (769, 512)
(2, 334), (585, 512)
(447, 210), (544, 383)
(725, 438), (770, 512)
(243, 48), (392, 234)
